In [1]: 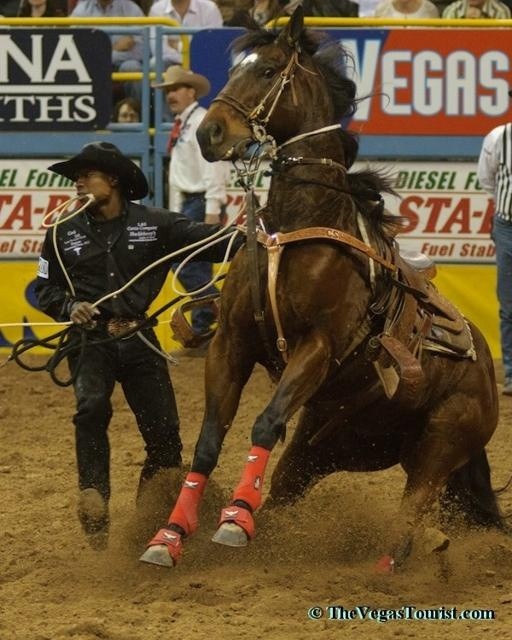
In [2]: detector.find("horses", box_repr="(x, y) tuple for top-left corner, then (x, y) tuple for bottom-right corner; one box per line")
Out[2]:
(140, 5), (512, 575)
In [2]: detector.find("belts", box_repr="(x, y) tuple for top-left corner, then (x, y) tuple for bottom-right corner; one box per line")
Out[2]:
(85, 316), (157, 340)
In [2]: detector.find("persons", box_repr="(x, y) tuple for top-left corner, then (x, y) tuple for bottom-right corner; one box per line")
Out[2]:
(477, 122), (511, 401)
(1, 1), (510, 125)
(151, 63), (230, 345)
(25, 140), (246, 549)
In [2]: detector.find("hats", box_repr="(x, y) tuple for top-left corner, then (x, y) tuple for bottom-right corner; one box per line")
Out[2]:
(47, 142), (148, 201)
(152, 65), (210, 98)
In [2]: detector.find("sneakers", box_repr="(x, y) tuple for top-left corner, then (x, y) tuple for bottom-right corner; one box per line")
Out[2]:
(77, 489), (109, 551)
